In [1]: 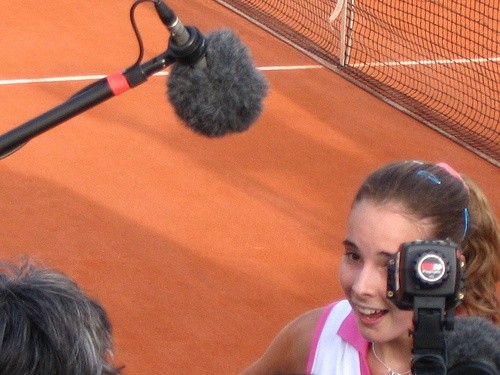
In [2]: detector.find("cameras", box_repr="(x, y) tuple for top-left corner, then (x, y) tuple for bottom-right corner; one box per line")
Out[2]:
(387, 238), (468, 375)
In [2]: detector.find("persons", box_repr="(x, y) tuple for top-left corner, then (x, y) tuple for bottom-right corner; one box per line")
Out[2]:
(0, 256), (125, 375)
(239, 160), (500, 375)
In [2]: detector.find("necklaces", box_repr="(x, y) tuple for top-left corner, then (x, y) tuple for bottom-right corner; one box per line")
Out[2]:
(372, 342), (411, 375)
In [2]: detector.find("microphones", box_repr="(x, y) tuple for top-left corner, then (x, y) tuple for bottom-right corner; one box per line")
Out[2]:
(446, 316), (500, 375)
(152, 0), (269, 138)
